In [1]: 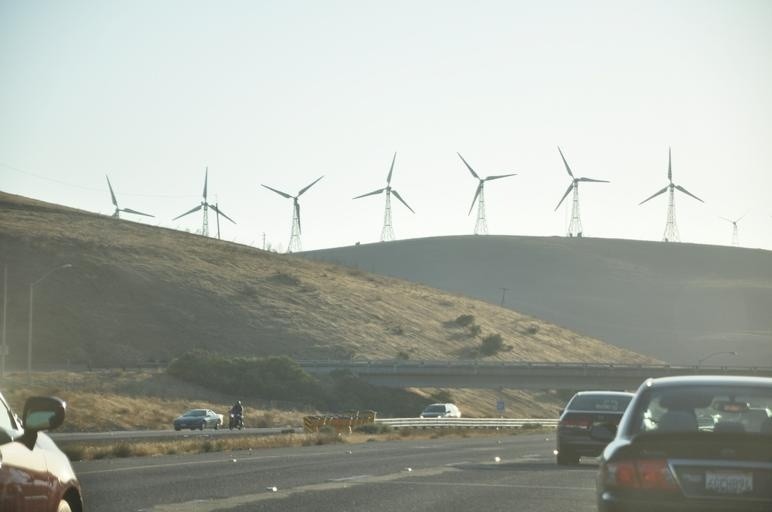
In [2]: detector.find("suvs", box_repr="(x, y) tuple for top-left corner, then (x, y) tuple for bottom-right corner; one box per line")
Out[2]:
(421, 402), (462, 428)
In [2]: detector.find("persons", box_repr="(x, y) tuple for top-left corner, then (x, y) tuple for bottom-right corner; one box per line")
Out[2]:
(230, 400), (243, 430)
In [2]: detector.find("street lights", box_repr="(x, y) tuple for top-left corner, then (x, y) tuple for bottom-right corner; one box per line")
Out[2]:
(698, 350), (740, 370)
(26, 262), (73, 389)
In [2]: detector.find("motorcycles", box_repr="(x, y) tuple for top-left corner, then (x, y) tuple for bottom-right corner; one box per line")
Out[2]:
(228, 409), (250, 432)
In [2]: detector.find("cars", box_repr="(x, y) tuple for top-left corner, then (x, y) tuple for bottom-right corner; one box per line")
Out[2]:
(1, 385), (91, 511)
(551, 389), (655, 468)
(594, 373), (772, 511)
(173, 406), (224, 432)
